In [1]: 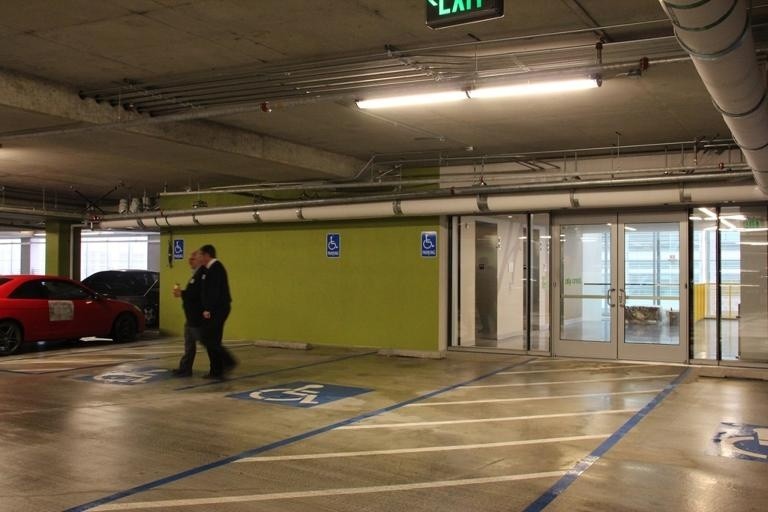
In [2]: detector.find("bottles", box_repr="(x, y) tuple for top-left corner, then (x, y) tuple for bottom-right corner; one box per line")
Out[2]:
(174, 284), (179, 291)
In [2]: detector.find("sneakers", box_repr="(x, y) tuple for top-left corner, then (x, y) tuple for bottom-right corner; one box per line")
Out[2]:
(203, 357), (235, 377)
(172, 369), (192, 376)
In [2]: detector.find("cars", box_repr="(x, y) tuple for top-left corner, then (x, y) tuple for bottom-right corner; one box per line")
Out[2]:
(1, 275), (147, 356)
(83, 270), (159, 325)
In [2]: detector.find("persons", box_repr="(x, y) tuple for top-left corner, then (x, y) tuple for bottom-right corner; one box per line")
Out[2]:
(172, 247), (238, 379)
(197, 245), (231, 381)
